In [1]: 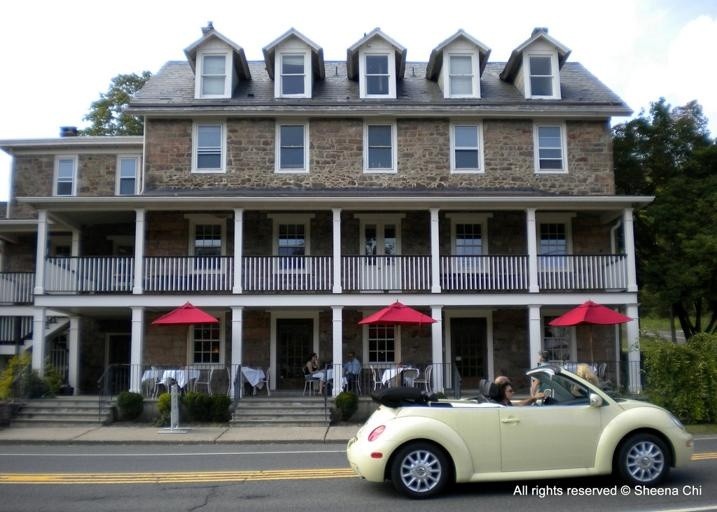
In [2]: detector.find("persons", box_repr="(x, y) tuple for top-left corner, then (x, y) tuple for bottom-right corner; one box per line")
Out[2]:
(342, 351), (360, 393)
(305, 352), (324, 395)
(494, 375), (545, 407)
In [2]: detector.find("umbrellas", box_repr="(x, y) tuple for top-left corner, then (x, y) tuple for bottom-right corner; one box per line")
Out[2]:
(150, 302), (220, 396)
(549, 300), (634, 367)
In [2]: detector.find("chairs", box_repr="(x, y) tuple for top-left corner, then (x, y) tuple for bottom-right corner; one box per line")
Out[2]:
(128, 360), (613, 405)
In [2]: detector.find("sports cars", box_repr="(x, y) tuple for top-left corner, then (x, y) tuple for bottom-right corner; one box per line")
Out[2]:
(347, 362), (693, 500)
